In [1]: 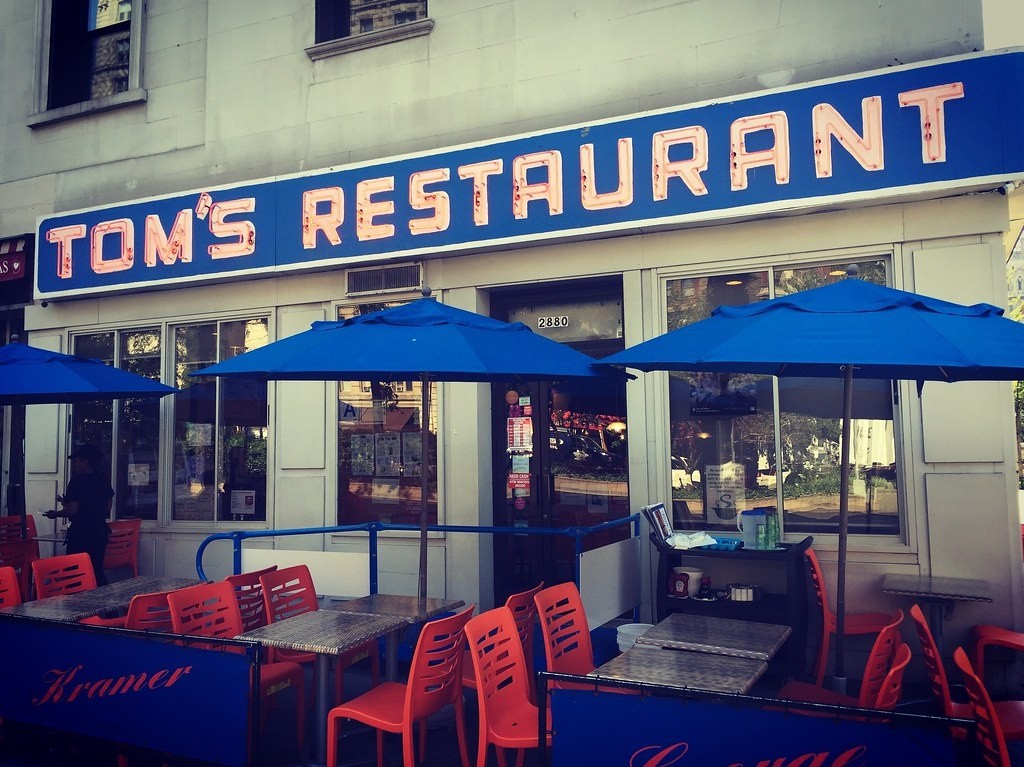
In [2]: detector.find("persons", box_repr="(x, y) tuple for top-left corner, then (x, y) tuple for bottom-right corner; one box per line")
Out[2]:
(42, 444), (115, 592)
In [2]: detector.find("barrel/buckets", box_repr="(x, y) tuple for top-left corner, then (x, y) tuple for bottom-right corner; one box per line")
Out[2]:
(615, 623), (654, 654)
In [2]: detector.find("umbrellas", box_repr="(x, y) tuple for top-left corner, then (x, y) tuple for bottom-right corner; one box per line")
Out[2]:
(188, 290), (636, 631)
(591, 265), (1024, 694)
(0, 333), (180, 540)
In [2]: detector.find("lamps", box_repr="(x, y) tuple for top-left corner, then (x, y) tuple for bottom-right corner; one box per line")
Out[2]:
(997, 180), (1023, 196)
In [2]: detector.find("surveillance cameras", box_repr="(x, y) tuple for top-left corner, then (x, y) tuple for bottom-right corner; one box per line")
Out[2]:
(40, 300), (49, 308)
(998, 182), (1016, 195)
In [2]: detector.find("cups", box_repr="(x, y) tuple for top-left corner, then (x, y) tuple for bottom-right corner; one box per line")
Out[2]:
(753, 523), (766, 549)
(673, 566), (703, 596)
(766, 512), (780, 548)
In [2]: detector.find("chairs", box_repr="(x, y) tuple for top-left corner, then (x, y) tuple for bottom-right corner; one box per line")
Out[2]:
(328, 580), (645, 767)
(0, 512), (379, 767)
(760, 547), (1024, 767)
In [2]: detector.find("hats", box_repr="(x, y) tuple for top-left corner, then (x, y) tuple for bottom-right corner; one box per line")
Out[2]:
(67, 444), (99, 459)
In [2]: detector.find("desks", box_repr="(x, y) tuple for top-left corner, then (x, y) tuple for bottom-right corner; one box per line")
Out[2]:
(32, 532), (69, 541)
(68, 575), (205, 606)
(0, 594), (130, 624)
(318, 594), (466, 739)
(882, 572), (994, 706)
(233, 610), (407, 767)
(587, 644), (768, 695)
(636, 613), (792, 663)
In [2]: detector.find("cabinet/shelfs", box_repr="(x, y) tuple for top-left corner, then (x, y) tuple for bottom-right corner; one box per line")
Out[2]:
(650, 532), (814, 650)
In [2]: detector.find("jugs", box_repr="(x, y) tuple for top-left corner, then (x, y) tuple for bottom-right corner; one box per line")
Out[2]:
(737, 511), (767, 549)
